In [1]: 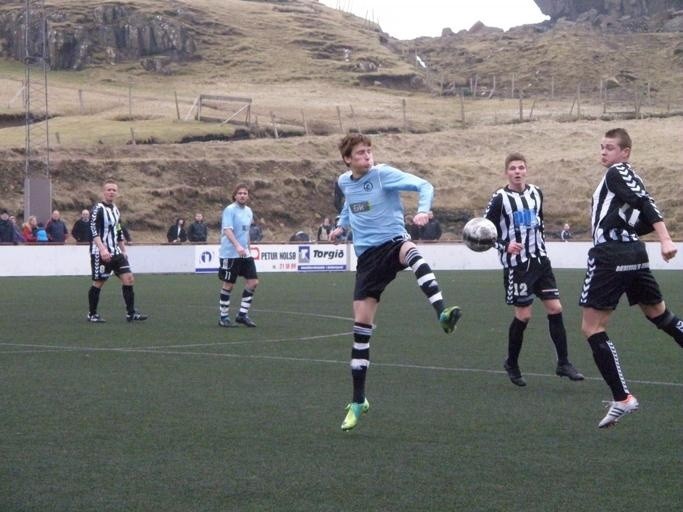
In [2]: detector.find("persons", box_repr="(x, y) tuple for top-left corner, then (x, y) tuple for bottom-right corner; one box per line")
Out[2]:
(289, 231), (308, 244)
(404, 215), (419, 244)
(72, 209), (92, 245)
(560, 223), (574, 240)
(317, 217), (336, 244)
(21, 216), (37, 245)
(218, 183), (259, 328)
(482, 152), (583, 386)
(166, 218), (187, 246)
(248, 215), (264, 244)
(330, 216), (352, 244)
(120, 222), (132, 245)
(32, 222), (48, 245)
(185, 213), (208, 245)
(44, 210), (68, 245)
(8, 215), (24, 242)
(87, 180), (148, 323)
(577, 129), (682, 428)
(418, 210), (441, 243)
(0, 208), (16, 245)
(328, 133), (462, 430)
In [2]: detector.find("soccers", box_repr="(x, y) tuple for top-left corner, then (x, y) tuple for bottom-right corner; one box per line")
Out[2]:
(463, 217), (497, 251)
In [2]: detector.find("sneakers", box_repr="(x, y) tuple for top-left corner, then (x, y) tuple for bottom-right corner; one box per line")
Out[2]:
(556, 362), (585, 380)
(126, 311), (148, 321)
(437, 306), (461, 334)
(341, 397), (369, 430)
(235, 313), (256, 327)
(503, 359), (527, 386)
(218, 317), (238, 327)
(599, 395), (640, 428)
(87, 312), (106, 323)
